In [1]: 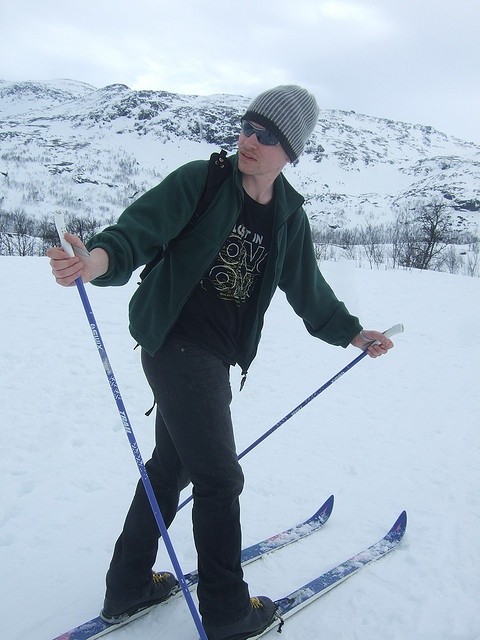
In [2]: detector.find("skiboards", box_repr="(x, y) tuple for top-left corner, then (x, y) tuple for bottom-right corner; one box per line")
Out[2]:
(54, 495), (407, 640)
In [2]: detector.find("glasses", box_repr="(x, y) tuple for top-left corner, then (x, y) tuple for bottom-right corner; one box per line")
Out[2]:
(239, 118), (280, 149)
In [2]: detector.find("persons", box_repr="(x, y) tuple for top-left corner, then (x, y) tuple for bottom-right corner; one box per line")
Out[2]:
(46, 83), (394, 640)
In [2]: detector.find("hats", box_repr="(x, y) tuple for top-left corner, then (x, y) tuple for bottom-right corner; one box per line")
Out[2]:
(241, 84), (320, 163)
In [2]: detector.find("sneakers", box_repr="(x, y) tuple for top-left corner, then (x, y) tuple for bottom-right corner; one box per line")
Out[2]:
(203, 595), (277, 640)
(100, 572), (177, 624)
